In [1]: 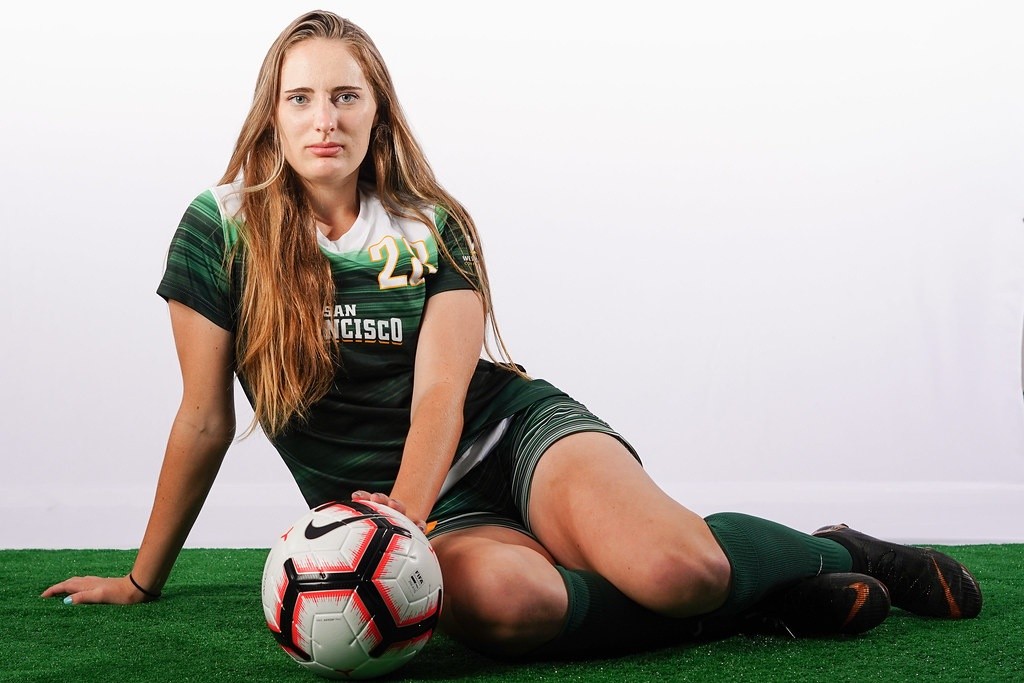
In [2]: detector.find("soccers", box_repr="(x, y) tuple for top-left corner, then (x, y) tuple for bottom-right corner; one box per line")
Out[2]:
(260, 499), (444, 683)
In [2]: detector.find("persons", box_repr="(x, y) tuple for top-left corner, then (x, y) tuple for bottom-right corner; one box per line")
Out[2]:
(40, 7), (981, 659)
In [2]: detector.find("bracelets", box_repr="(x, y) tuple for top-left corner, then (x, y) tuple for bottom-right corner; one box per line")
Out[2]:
(129, 574), (161, 598)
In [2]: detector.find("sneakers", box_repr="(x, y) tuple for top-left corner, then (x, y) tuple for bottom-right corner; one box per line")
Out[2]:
(741, 572), (892, 641)
(809, 522), (982, 618)
